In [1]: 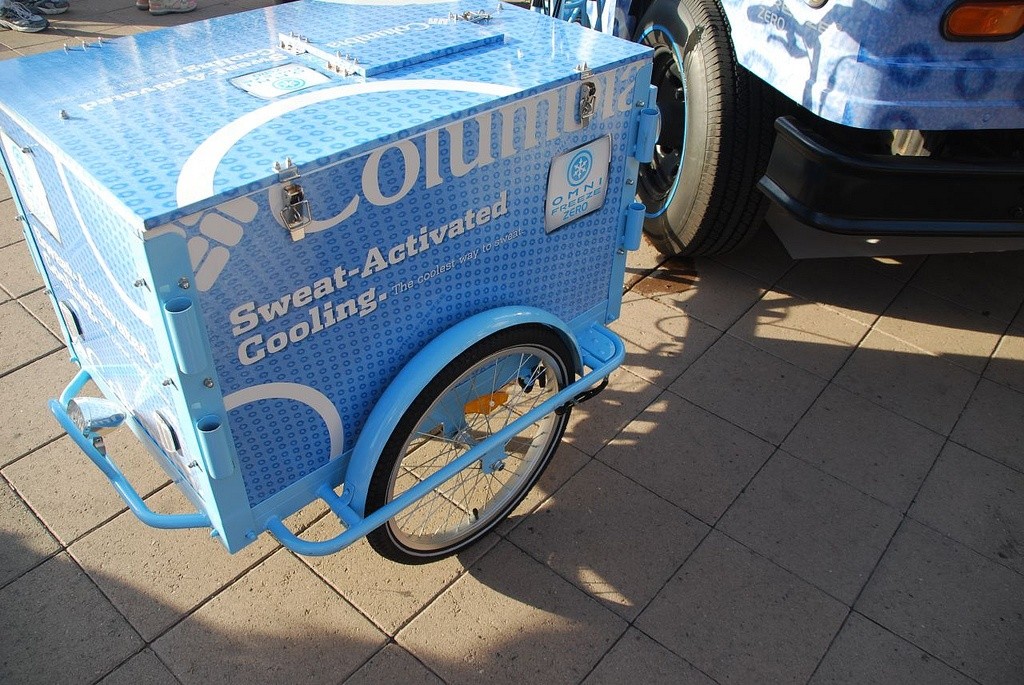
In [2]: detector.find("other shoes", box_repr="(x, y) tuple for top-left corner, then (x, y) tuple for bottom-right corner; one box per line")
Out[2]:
(136, 0), (149, 10)
(149, 0), (197, 15)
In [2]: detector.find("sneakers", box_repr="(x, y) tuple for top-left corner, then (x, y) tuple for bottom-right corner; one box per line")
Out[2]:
(18, 0), (69, 15)
(0, 0), (50, 33)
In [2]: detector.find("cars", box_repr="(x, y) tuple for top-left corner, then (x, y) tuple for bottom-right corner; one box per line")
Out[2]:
(616, 0), (1024, 264)
